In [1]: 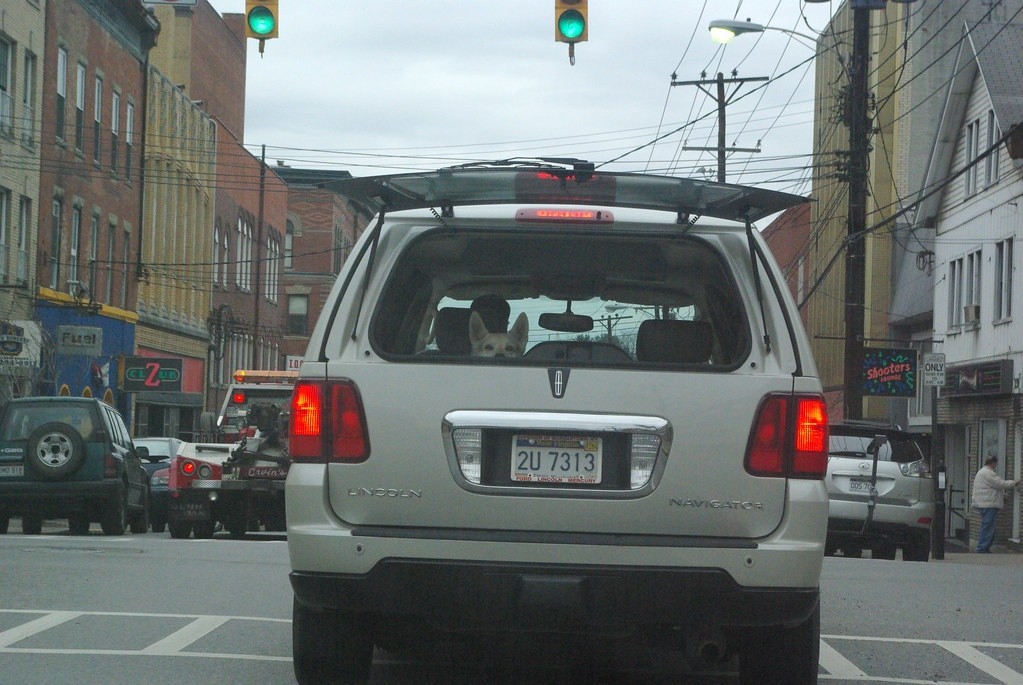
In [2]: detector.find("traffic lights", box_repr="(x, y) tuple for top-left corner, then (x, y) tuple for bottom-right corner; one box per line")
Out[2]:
(245, 0), (279, 58)
(554, 0), (589, 65)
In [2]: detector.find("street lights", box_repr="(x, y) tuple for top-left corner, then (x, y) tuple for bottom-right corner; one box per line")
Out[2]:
(708, 21), (850, 86)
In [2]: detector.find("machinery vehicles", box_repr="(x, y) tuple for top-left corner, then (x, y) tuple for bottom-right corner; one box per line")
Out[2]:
(165, 369), (298, 539)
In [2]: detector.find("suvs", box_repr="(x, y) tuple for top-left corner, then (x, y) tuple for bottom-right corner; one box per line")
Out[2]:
(824, 425), (935, 561)
(0, 398), (151, 535)
(285, 154), (831, 684)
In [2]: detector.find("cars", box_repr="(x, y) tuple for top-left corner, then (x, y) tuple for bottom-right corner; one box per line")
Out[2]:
(68, 438), (184, 532)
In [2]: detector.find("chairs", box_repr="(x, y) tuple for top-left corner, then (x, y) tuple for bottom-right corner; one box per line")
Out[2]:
(636, 320), (714, 364)
(435, 308), (508, 354)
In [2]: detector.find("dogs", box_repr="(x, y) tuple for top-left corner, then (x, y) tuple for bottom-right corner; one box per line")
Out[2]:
(91, 362), (110, 387)
(468, 311), (530, 358)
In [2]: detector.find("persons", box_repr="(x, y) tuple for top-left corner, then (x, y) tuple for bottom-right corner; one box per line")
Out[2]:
(470, 294), (511, 334)
(972, 456), (1021, 553)
